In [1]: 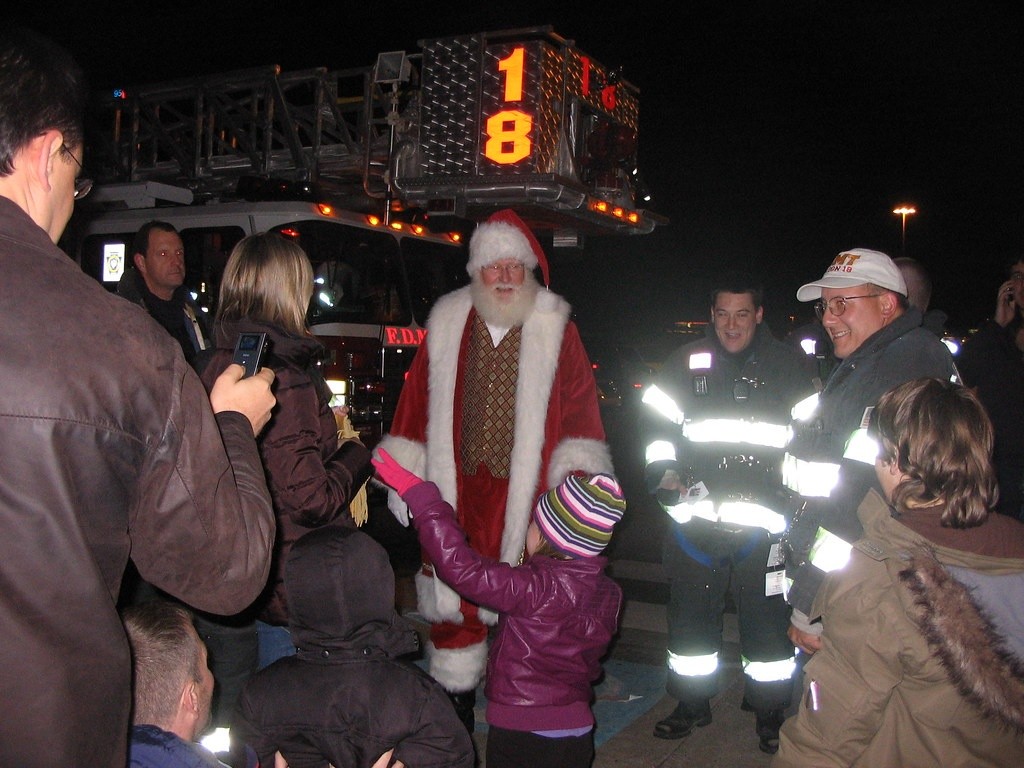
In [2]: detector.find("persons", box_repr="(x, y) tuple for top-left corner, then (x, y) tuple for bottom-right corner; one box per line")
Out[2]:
(1, 24), (478, 768)
(636, 246), (1024, 767)
(372, 209), (628, 767)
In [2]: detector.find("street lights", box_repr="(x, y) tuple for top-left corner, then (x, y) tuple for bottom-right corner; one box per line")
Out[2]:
(893, 208), (917, 254)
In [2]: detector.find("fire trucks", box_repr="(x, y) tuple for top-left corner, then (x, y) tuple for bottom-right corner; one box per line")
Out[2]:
(61, 23), (655, 448)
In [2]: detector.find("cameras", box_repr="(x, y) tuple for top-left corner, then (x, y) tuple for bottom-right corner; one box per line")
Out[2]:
(232, 332), (270, 381)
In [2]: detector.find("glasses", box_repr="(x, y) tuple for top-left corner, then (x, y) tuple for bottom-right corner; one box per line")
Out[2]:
(62, 143), (94, 200)
(482, 264), (524, 272)
(814, 293), (880, 320)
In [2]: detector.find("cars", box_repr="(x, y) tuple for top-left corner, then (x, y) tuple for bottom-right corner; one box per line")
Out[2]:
(582, 328), (681, 411)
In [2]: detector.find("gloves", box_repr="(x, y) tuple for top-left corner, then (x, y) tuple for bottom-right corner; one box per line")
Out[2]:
(372, 448), (424, 497)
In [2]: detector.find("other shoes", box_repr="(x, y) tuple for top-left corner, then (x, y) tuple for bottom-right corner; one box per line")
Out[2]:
(756, 707), (785, 754)
(653, 698), (712, 739)
(450, 688), (475, 736)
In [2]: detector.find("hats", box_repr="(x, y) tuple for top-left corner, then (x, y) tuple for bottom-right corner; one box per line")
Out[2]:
(796, 247), (907, 302)
(466, 208), (558, 313)
(534, 473), (626, 559)
